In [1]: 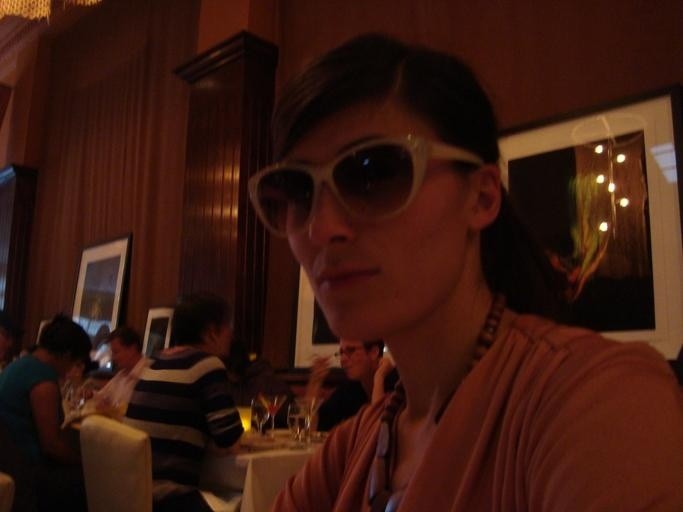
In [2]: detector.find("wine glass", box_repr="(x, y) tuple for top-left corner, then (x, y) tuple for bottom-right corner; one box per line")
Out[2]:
(251, 392), (325, 443)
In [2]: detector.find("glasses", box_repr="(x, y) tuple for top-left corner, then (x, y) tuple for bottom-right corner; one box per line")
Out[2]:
(333, 346), (364, 360)
(246, 132), (485, 238)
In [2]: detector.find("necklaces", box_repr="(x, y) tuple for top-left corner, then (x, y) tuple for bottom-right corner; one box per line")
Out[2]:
(368, 291), (508, 512)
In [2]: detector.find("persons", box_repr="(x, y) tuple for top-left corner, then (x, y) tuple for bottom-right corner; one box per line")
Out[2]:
(0, 291), (244, 512)
(267, 33), (683, 512)
(301, 335), (399, 432)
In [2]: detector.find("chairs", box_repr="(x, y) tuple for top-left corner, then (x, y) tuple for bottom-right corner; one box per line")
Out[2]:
(80, 415), (153, 511)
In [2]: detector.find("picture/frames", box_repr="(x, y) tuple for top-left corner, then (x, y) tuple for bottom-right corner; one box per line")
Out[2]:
(70, 231), (174, 364)
(496, 84), (681, 379)
(289, 264), (341, 368)
